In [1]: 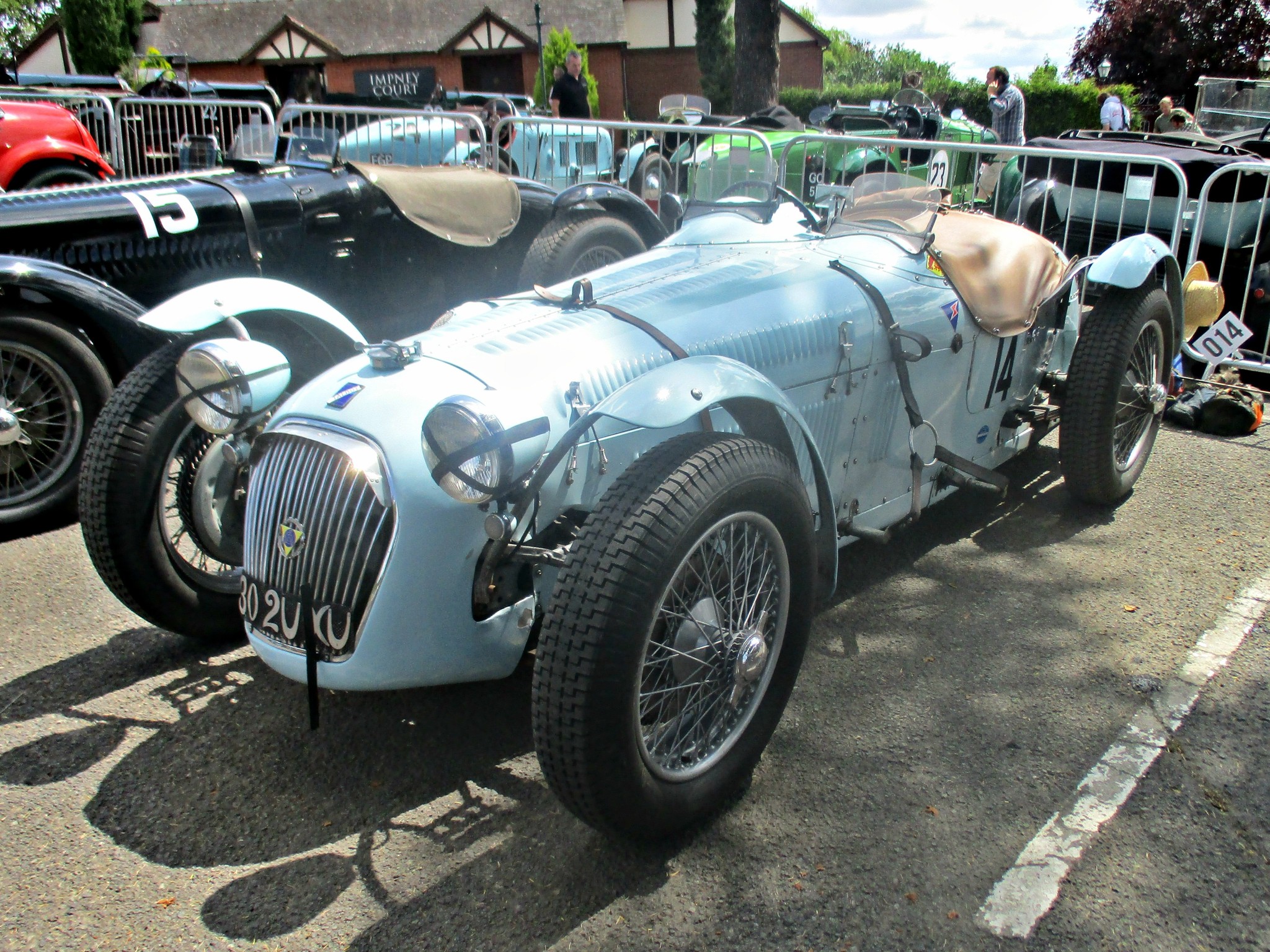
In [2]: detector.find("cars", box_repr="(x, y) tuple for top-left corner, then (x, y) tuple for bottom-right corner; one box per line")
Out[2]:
(1, 73), (1269, 394)
(0, 98), (117, 194)
(0, 122), (671, 545)
(78, 169), (1188, 850)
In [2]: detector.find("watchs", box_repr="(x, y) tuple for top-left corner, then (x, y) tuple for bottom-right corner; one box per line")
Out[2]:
(988, 94), (994, 98)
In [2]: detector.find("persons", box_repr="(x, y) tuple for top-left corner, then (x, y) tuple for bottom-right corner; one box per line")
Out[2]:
(1170, 112), (1203, 135)
(977, 66), (1024, 200)
(1153, 97), (1193, 133)
(549, 66), (564, 105)
(550, 52), (593, 120)
(1097, 92), (1130, 131)
(283, 77), (317, 123)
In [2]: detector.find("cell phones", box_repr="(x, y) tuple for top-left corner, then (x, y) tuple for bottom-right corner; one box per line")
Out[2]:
(996, 77), (1002, 85)
